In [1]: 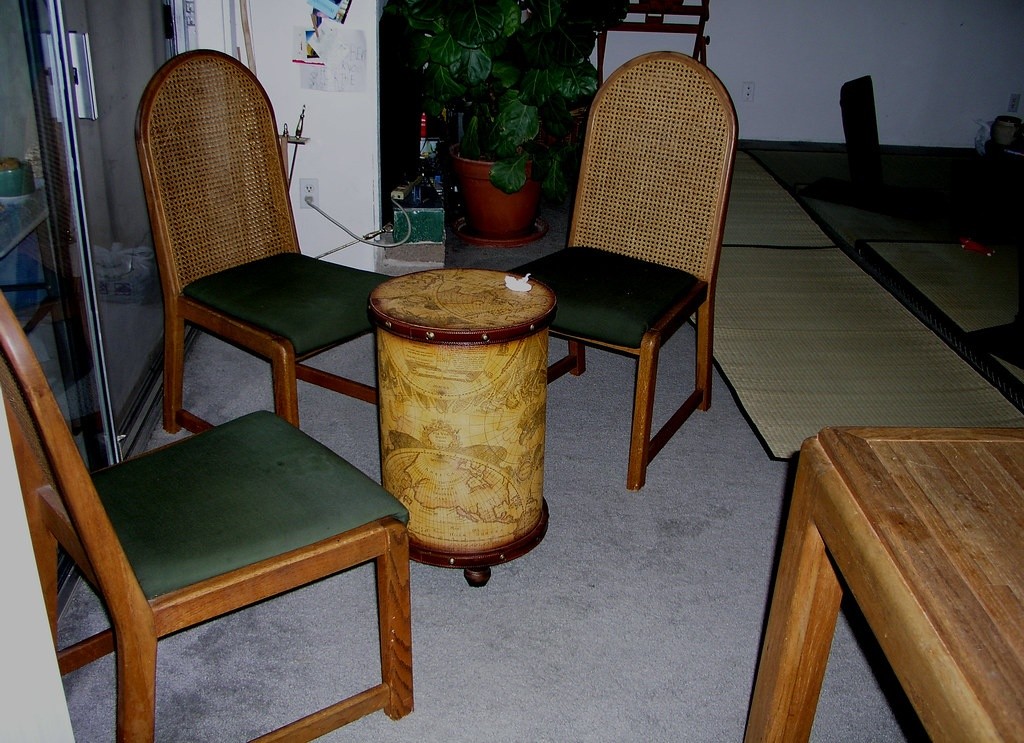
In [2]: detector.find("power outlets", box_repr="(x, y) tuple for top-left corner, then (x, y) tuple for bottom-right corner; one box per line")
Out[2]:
(1007, 94), (1021, 112)
(742, 82), (755, 102)
(299, 178), (319, 210)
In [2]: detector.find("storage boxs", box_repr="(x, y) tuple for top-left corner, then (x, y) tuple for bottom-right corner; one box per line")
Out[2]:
(392, 208), (445, 244)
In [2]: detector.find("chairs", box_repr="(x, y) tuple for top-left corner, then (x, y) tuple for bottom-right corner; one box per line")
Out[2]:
(134, 49), (397, 436)
(0, 289), (414, 743)
(506, 50), (740, 493)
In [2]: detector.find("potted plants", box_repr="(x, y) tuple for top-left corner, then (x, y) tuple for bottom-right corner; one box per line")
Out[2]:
(379, 0), (631, 249)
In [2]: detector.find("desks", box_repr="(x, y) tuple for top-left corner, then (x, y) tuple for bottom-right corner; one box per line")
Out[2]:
(743, 425), (1024, 743)
(368, 268), (559, 588)
